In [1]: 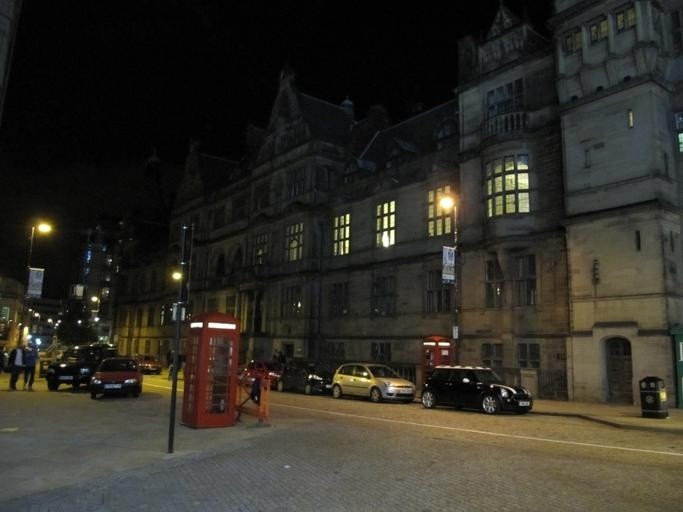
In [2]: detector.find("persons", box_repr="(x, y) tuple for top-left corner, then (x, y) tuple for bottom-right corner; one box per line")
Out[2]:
(25, 345), (39, 390)
(7, 343), (25, 390)
(168, 351), (174, 378)
(278, 351), (285, 363)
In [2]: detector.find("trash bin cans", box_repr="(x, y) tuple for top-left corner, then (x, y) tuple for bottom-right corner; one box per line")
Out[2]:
(639, 376), (668, 418)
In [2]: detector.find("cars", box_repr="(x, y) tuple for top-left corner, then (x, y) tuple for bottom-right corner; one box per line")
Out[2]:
(239, 358), (285, 392)
(89, 355), (144, 400)
(331, 361), (419, 405)
(275, 360), (333, 396)
(419, 363), (535, 417)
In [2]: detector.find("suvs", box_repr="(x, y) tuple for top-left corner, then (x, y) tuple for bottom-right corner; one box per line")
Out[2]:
(45, 344), (119, 391)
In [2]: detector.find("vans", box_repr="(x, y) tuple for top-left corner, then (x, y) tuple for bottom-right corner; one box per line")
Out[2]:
(137, 352), (162, 375)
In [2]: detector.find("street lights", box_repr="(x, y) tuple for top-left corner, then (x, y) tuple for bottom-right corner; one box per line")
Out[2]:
(18, 222), (52, 341)
(437, 194), (460, 365)
